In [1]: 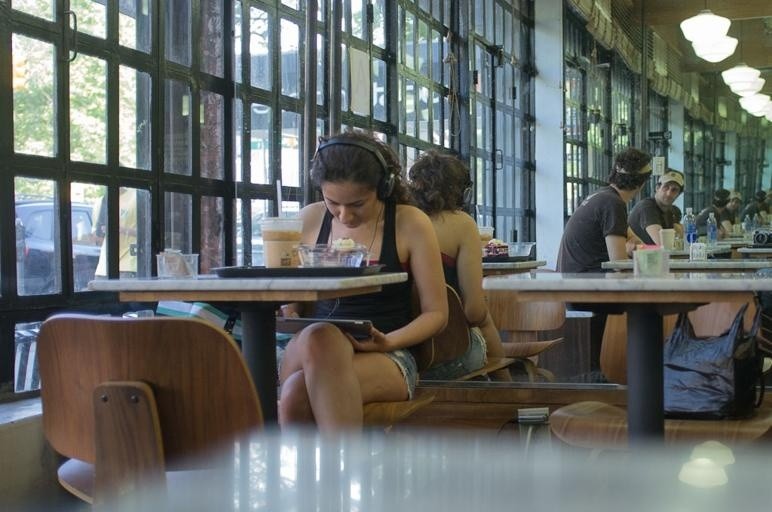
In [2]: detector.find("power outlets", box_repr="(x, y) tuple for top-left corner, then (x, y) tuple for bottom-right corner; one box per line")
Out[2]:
(517, 407), (550, 442)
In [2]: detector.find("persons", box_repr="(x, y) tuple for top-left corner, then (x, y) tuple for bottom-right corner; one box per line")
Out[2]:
(96, 157), (140, 281)
(693, 188), (731, 246)
(724, 190), (742, 225)
(555, 148), (653, 316)
(405, 149), (514, 384)
(739, 189), (766, 224)
(280, 132), (448, 436)
(627, 168), (685, 251)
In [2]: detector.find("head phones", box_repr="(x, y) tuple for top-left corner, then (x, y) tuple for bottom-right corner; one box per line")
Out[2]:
(463, 173), (474, 204)
(311, 137), (396, 201)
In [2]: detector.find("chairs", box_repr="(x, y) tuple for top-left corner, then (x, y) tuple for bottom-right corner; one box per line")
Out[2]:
(36, 312), (264, 512)
(438, 284), (530, 385)
(487, 292), (564, 386)
(549, 291), (772, 443)
(325, 281), (435, 423)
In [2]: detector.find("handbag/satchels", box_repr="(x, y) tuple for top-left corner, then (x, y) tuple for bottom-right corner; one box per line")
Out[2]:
(662, 304), (765, 421)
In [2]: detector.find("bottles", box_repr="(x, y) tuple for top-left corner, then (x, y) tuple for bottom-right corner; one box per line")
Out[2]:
(708, 212), (718, 245)
(754, 214), (758, 235)
(746, 215), (752, 245)
(684, 207), (699, 254)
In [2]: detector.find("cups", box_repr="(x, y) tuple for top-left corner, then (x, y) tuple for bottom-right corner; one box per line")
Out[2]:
(657, 229), (676, 249)
(257, 216), (304, 268)
(478, 227), (494, 251)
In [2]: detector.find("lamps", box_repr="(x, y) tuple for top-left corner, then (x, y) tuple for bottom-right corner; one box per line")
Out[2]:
(721, 21), (766, 97)
(680, 0), (738, 63)
(739, 94), (772, 123)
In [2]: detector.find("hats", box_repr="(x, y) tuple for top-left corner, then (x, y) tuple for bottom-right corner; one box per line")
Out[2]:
(715, 194), (730, 200)
(616, 162), (652, 174)
(659, 171), (684, 186)
(730, 193), (743, 200)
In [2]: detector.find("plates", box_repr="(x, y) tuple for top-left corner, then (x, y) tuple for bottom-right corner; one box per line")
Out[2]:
(481, 254), (535, 262)
(208, 264), (388, 278)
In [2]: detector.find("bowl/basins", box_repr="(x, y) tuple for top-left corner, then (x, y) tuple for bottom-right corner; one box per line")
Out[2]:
(292, 244), (370, 268)
(507, 241), (537, 257)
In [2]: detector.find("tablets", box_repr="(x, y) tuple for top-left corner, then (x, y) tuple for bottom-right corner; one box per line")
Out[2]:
(276, 316), (372, 339)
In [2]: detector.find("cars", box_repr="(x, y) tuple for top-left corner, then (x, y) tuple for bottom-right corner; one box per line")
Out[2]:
(236, 210), (299, 254)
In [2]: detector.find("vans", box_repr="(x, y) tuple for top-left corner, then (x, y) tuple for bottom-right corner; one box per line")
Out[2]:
(15, 200), (101, 292)
